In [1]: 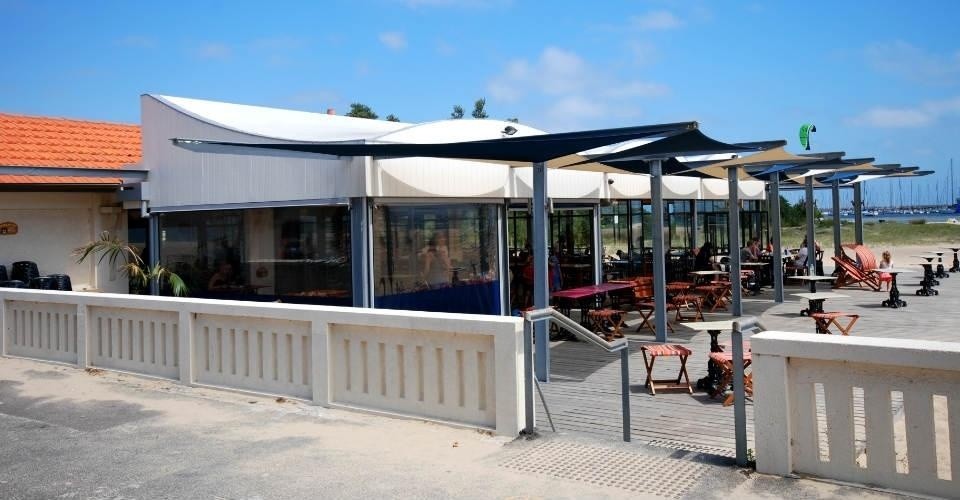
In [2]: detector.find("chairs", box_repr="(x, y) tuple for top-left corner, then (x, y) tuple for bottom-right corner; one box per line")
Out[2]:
(742, 240), (890, 312)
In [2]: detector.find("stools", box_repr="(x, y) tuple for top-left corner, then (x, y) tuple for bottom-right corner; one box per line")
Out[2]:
(520, 269), (753, 340)
(639, 309), (864, 406)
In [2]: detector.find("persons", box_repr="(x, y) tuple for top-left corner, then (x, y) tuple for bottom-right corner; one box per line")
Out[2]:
(785, 239), (808, 267)
(878, 251), (895, 292)
(751, 237), (767, 278)
(766, 236), (774, 252)
(739, 240), (759, 262)
(693, 242), (719, 281)
(610, 250), (628, 281)
(548, 247), (564, 291)
(279, 231), (290, 259)
(805, 234), (821, 255)
(207, 262), (234, 290)
(418, 229), (450, 276)
(521, 255), (534, 308)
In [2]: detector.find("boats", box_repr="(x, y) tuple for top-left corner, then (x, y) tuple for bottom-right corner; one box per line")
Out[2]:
(820, 158), (956, 216)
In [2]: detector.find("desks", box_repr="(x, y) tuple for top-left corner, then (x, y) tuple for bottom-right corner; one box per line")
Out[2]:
(866, 241), (960, 307)
